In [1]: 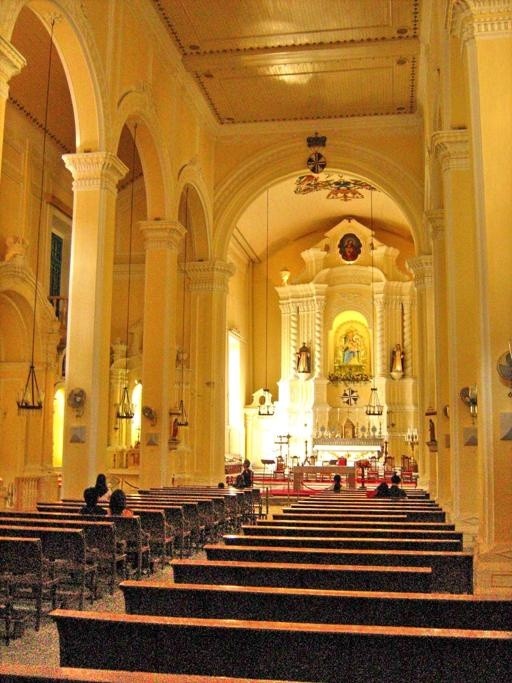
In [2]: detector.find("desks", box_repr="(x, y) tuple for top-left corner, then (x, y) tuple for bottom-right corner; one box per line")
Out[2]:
(291, 465), (356, 490)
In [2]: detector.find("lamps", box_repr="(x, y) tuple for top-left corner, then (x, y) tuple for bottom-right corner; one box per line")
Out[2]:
(114, 124), (135, 419)
(16, 20), (57, 410)
(258, 190), (274, 416)
(175, 188), (188, 426)
(366, 189), (383, 415)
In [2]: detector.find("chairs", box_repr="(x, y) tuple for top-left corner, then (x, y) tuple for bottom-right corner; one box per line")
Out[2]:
(367, 453), (414, 483)
(271, 455), (346, 482)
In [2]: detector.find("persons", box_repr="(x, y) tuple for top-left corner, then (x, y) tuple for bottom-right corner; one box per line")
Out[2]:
(218, 482), (224, 488)
(242, 459), (252, 487)
(374, 482), (391, 498)
(327, 474), (345, 493)
(390, 343), (404, 372)
(106, 489), (133, 517)
(296, 342), (311, 373)
(79, 486), (108, 515)
(233, 475), (246, 489)
(94, 473), (112, 503)
(388, 475), (408, 497)
(343, 239), (357, 259)
